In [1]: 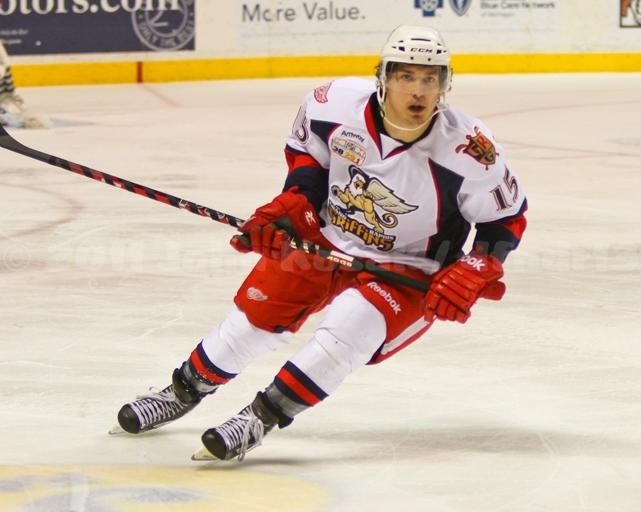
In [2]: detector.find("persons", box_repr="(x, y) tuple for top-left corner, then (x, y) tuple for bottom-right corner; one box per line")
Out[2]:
(117, 24), (530, 467)
(0, 38), (26, 130)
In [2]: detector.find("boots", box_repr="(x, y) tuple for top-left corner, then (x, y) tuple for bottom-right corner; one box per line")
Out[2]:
(118, 364), (217, 436)
(204, 388), (286, 457)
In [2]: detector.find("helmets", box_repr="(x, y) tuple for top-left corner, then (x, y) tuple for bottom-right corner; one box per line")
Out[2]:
(377, 23), (453, 100)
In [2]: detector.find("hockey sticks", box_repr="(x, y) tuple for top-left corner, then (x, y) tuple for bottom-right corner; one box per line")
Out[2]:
(0, 122), (430, 294)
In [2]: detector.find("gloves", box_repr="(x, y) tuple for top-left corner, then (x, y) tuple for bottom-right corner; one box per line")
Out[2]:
(229, 187), (312, 262)
(422, 247), (507, 327)
